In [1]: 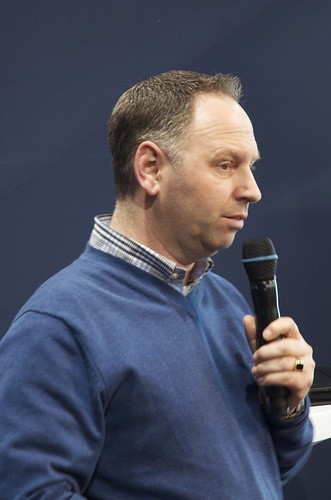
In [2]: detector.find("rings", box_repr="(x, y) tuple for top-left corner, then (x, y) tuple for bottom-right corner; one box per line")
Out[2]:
(294, 355), (303, 372)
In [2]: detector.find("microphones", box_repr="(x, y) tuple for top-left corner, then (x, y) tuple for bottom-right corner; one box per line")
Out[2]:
(241, 237), (290, 420)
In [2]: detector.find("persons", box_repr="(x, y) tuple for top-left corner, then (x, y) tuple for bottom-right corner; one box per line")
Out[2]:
(0, 71), (316, 500)
